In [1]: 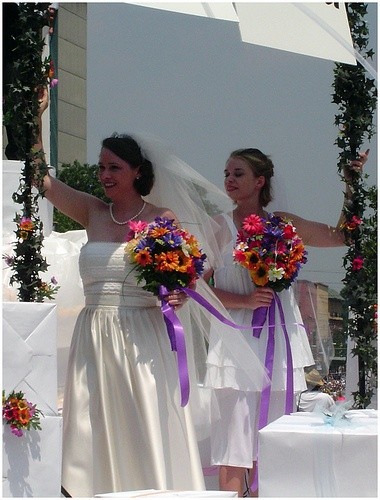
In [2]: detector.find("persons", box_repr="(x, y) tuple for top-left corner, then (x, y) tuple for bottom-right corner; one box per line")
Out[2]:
(297, 368), (335, 413)
(29, 73), (200, 492)
(198, 147), (371, 495)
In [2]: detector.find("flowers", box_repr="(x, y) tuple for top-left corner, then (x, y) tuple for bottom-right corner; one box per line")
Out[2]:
(232, 212), (308, 337)
(121, 217), (207, 353)
(2, 389), (45, 437)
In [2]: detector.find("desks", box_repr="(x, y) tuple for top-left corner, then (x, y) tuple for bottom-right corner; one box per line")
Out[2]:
(258, 409), (378, 497)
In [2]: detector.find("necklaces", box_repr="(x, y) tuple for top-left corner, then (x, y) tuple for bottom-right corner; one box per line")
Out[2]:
(109, 201), (147, 225)
